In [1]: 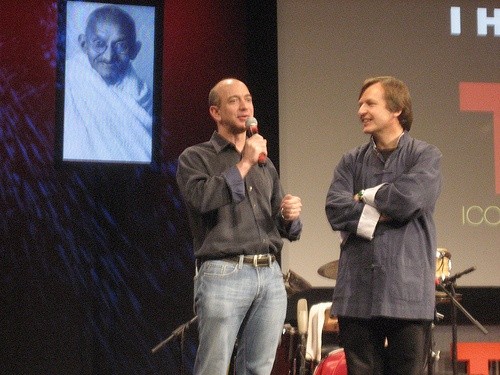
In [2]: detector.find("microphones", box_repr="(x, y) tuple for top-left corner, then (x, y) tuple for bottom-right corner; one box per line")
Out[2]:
(245, 117), (267, 167)
(297, 299), (308, 362)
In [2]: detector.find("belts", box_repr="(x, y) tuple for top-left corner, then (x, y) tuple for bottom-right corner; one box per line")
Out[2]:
(219, 256), (276, 265)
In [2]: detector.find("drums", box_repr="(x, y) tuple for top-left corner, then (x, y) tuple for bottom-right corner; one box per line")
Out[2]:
(277, 324), (301, 363)
(434, 248), (453, 278)
(312, 348), (347, 375)
(323, 307), (341, 333)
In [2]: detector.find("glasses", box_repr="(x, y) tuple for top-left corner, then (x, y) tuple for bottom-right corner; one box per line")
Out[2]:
(88, 40), (136, 53)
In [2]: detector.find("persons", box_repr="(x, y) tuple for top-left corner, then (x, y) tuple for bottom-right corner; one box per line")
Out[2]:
(65, 4), (151, 162)
(176, 79), (301, 375)
(327, 76), (442, 375)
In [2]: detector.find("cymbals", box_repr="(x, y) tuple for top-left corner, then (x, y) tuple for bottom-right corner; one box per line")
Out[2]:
(316, 259), (340, 280)
(431, 287), (462, 305)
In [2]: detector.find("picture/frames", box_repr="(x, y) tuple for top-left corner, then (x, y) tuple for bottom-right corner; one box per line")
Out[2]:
(55, 0), (164, 174)
